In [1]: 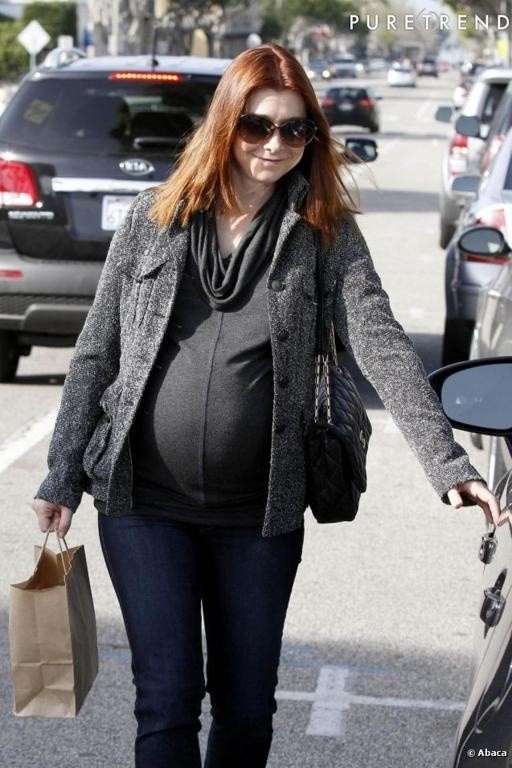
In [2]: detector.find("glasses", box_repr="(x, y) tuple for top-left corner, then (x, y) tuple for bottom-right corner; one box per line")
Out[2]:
(237, 114), (318, 147)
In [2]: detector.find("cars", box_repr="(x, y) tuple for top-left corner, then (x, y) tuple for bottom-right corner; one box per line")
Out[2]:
(413, 54), (439, 79)
(427, 51), (511, 767)
(385, 61), (418, 88)
(318, 85), (386, 135)
(329, 52), (361, 81)
(306, 57), (331, 80)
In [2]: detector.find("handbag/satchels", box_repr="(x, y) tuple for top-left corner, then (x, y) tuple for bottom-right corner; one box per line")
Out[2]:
(304, 363), (372, 523)
(10, 528), (98, 718)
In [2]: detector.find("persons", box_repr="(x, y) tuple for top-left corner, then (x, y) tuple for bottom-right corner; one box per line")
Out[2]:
(31, 42), (501, 768)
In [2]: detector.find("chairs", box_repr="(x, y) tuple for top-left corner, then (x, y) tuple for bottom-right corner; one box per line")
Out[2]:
(129, 111), (193, 150)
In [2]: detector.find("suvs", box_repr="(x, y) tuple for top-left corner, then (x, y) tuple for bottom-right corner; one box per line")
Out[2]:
(2, 25), (378, 383)
